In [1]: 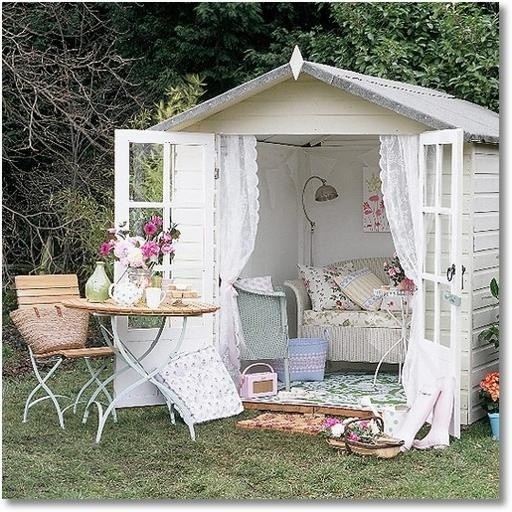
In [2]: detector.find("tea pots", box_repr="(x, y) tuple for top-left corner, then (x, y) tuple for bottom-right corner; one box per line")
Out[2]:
(109, 279), (147, 308)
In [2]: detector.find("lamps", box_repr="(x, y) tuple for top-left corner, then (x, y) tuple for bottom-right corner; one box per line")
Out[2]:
(301, 176), (339, 227)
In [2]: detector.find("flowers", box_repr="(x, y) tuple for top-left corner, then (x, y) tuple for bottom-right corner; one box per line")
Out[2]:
(383, 249), (405, 283)
(321, 416), (390, 444)
(100, 215), (181, 281)
(473, 372), (500, 414)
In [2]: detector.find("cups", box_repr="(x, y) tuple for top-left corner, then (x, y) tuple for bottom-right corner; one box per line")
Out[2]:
(146, 287), (167, 309)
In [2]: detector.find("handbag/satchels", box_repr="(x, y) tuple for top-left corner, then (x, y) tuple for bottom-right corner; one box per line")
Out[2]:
(9, 302), (93, 355)
(268, 336), (330, 384)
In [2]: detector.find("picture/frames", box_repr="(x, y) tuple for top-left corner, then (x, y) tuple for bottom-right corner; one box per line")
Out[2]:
(362, 166), (391, 233)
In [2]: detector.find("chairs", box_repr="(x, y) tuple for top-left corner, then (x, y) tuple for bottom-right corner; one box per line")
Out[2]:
(15, 273), (118, 430)
(232, 275), (290, 392)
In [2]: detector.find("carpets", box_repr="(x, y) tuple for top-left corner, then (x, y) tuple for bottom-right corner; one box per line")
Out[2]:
(241, 367), (407, 411)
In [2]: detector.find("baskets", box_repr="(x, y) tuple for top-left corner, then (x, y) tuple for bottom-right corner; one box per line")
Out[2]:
(324, 414), (406, 460)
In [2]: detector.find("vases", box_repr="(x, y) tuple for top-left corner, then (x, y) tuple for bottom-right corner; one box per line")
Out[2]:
(488, 413), (499, 440)
(126, 268), (154, 307)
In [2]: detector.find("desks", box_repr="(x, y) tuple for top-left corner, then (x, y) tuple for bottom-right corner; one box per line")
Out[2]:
(60, 297), (221, 444)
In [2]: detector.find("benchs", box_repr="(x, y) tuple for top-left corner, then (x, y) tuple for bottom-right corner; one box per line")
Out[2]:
(283, 256), (412, 365)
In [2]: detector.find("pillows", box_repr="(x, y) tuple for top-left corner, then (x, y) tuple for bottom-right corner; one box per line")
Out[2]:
(332, 261), (390, 311)
(236, 275), (275, 293)
(295, 264), (362, 311)
